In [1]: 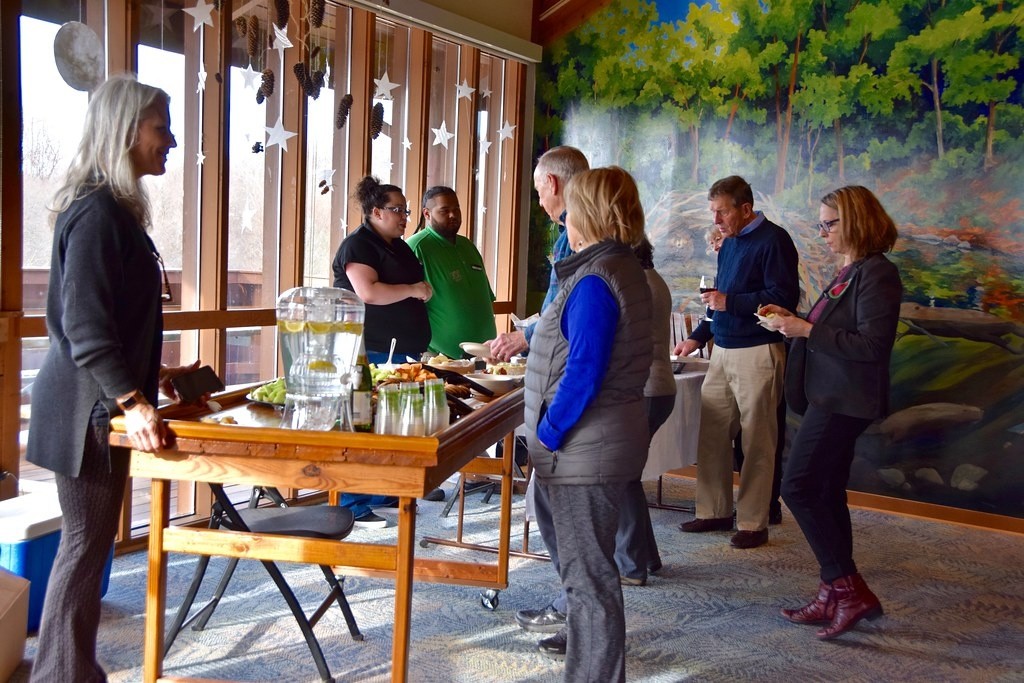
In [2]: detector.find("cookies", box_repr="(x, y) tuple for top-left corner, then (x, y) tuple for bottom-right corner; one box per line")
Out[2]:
(443, 383), (472, 398)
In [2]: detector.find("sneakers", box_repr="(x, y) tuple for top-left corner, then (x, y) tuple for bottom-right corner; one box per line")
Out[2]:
(424, 488), (445, 501)
(514, 605), (567, 632)
(464, 479), (494, 492)
(354, 512), (388, 528)
(646, 568), (656, 575)
(371, 500), (419, 513)
(678, 517), (733, 533)
(731, 528), (769, 548)
(619, 575), (646, 587)
(538, 629), (567, 662)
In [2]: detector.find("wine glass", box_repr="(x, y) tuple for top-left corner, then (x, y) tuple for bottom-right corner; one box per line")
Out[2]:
(699, 275), (717, 322)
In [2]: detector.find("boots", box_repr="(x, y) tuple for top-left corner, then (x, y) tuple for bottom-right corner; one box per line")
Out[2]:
(816, 573), (884, 641)
(780, 583), (840, 625)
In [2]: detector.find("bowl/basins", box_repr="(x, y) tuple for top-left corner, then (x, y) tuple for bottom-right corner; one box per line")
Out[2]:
(671, 362), (686, 374)
(464, 375), (515, 397)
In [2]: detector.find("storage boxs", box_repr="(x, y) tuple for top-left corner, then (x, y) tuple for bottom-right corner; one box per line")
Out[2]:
(0, 493), (114, 683)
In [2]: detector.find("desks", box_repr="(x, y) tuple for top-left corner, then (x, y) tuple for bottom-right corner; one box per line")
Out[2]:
(108, 378), (528, 683)
(420, 370), (707, 561)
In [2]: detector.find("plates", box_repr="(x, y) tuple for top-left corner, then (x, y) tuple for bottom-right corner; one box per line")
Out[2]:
(459, 342), (491, 359)
(753, 312), (770, 322)
(246, 393), (284, 406)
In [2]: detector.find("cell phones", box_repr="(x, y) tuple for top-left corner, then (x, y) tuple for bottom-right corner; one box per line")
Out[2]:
(170, 365), (224, 403)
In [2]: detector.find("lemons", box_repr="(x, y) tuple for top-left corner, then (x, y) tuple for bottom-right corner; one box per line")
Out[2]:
(308, 361), (336, 373)
(276, 319), (362, 335)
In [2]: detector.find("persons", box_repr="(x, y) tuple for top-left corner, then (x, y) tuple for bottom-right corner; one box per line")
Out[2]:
(758, 185), (901, 639)
(25, 75), (211, 683)
(333, 176), (432, 528)
(403, 186), (497, 500)
(482, 145), (590, 661)
(672, 176), (799, 548)
(524, 165), (650, 683)
(616, 231), (677, 586)
(705, 223), (788, 525)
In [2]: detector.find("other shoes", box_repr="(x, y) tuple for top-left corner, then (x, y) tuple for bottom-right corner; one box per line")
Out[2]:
(731, 502), (782, 525)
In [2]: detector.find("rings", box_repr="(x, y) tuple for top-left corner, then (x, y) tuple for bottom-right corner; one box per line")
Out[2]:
(497, 353), (502, 359)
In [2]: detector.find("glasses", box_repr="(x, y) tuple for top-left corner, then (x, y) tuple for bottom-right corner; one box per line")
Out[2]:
(158, 258), (172, 302)
(817, 218), (839, 233)
(383, 207), (412, 216)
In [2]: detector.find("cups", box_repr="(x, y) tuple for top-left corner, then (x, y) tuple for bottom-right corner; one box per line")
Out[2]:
(374, 384), (400, 436)
(422, 378), (450, 437)
(400, 381), (426, 436)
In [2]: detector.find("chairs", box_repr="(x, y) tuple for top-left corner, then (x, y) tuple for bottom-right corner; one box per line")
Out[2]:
(162, 483), (364, 683)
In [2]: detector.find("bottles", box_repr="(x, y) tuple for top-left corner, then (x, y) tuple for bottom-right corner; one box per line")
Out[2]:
(350, 328), (373, 432)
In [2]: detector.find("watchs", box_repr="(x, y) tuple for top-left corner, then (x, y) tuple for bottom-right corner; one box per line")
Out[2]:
(116, 388), (144, 411)
